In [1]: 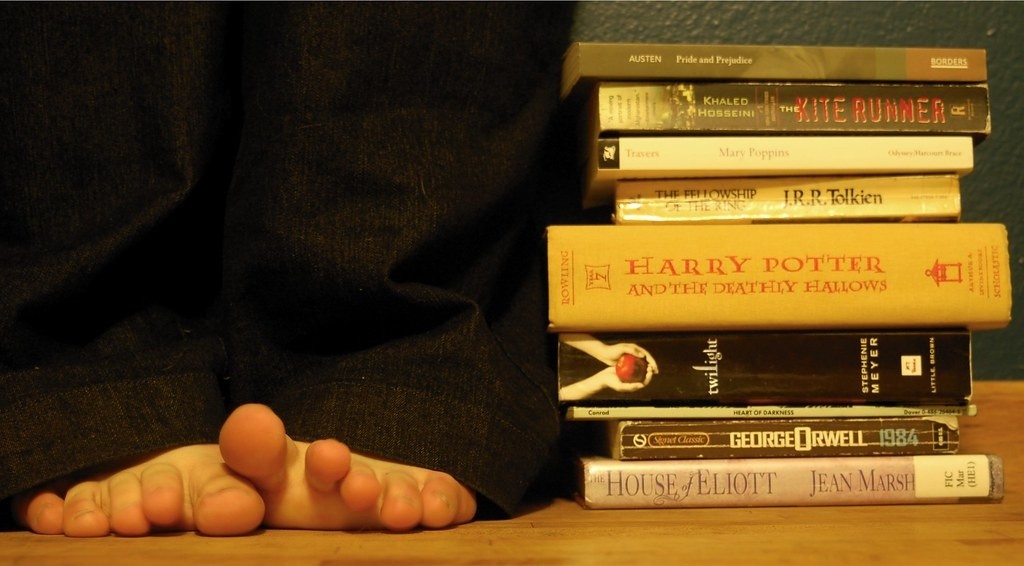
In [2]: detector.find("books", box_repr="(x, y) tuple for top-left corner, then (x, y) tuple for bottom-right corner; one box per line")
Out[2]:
(548, 225), (1016, 330)
(558, 331), (972, 404)
(560, 40), (988, 108)
(574, 454), (1004, 510)
(566, 405), (974, 419)
(602, 416), (964, 460)
(610, 173), (963, 227)
(581, 136), (973, 209)
(582, 82), (993, 150)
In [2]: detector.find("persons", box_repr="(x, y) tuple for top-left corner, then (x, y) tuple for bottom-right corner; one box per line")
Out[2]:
(0, 1), (580, 536)
